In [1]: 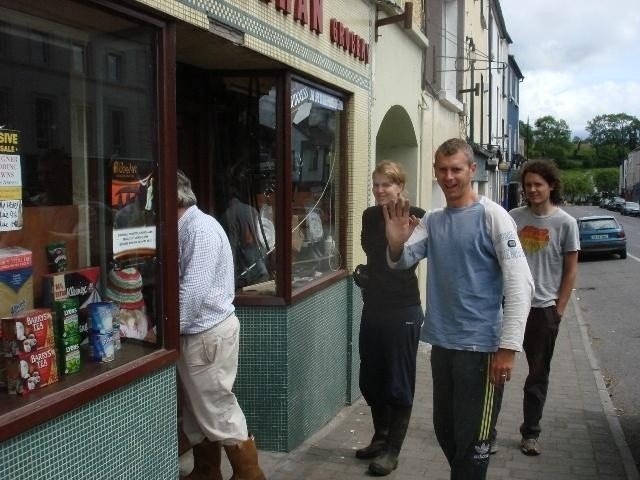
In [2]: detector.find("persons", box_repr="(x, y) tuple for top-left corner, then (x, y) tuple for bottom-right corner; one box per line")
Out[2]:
(488, 160), (582, 456)
(115, 184), (156, 228)
(223, 185), (276, 291)
(144, 169), (266, 480)
(354, 162), (426, 476)
(382, 137), (535, 479)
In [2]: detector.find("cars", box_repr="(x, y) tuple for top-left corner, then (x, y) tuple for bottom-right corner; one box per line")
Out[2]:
(597, 196), (640, 217)
(574, 214), (628, 261)
(290, 206), (328, 229)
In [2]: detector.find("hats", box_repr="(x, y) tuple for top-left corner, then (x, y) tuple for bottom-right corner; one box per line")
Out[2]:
(96, 266), (145, 310)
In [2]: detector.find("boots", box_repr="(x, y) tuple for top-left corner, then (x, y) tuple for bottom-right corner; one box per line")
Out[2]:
(225, 432), (267, 479)
(357, 406), (388, 459)
(181, 443), (223, 479)
(369, 406), (413, 476)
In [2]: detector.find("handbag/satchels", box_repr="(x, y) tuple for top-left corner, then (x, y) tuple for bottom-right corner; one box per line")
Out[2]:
(353, 263), (369, 288)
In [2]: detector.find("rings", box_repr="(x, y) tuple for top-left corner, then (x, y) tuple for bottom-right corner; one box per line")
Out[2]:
(500, 374), (506, 377)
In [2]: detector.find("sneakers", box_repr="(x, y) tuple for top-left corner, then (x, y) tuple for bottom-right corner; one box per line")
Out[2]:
(490, 439), (498, 453)
(520, 436), (540, 455)
